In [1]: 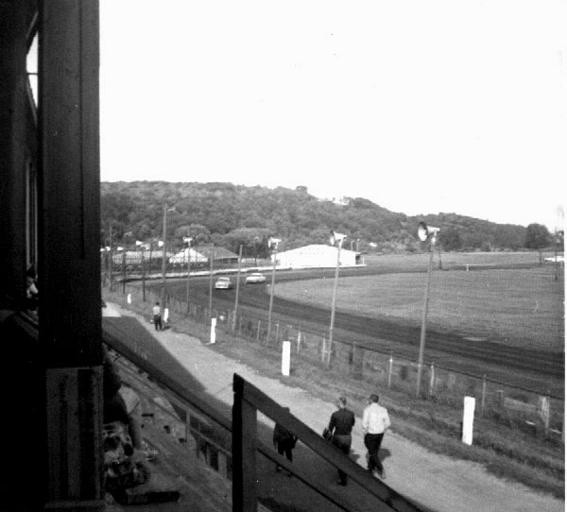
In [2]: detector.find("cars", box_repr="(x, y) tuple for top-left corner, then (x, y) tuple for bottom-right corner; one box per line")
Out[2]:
(214, 277), (233, 290)
(544, 255), (564, 263)
(246, 272), (266, 284)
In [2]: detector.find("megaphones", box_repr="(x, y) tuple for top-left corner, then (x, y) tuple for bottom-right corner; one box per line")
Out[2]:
(100, 240), (163, 252)
(182, 236), (192, 244)
(417, 222), (439, 243)
(267, 236), (281, 248)
(328, 230), (347, 246)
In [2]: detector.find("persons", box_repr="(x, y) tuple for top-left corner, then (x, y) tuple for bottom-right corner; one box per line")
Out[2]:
(327, 397), (355, 486)
(26, 262), (39, 297)
(151, 300), (162, 330)
(272, 406), (300, 476)
(361, 394), (391, 479)
(163, 305), (169, 328)
(103, 344), (158, 462)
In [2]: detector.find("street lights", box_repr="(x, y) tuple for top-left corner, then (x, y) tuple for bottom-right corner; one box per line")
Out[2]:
(266, 235), (281, 343)
(414, 223), (441, 398)
(182, 235), (191, 315)
(325, 230), (351, 369)
(99, 238), (169, 309)
(554, 239), (562, 281)
